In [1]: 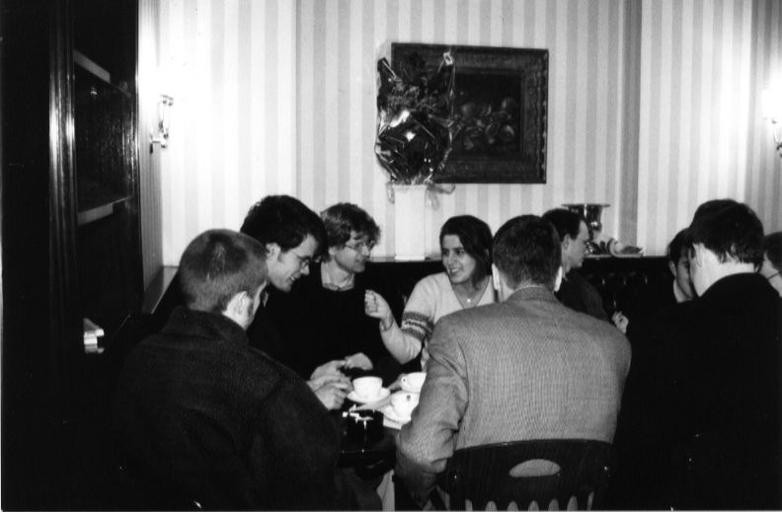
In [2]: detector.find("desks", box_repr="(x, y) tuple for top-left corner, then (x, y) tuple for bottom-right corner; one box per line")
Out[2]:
(326, 366), (402, 474)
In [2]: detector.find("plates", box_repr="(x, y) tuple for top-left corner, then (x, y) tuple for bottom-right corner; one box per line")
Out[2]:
(347, 387), (391, 405)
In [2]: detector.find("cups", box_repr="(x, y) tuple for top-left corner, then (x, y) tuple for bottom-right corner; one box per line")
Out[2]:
(352, 376), (383, 398)
(389, 391), (417, 419)
(402, 372), (427, 398)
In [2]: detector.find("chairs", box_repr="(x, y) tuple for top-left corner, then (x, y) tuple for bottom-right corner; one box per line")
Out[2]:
(441, 439), (616, 511)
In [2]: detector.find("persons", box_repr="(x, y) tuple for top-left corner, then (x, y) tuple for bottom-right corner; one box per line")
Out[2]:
(542, 201), (781, 511)
(109, 194), (497, 512)
(393, 215), (631, 510)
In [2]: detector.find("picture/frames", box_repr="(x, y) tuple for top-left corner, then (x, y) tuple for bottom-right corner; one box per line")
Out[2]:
(389, 42), (550, 184)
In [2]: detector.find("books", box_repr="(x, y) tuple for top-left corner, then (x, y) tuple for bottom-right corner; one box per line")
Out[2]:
(80, 314), (105, 357)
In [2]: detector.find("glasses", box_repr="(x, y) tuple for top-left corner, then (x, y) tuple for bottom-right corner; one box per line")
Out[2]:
(344, 240), (376, 250)
(292, 253), (312, 267)
